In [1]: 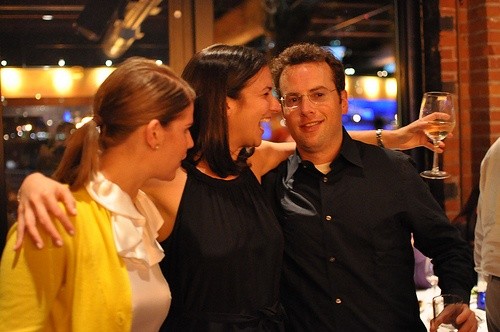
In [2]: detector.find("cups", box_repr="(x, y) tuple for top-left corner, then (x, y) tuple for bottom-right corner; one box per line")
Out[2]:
(432, 293), (463, 332)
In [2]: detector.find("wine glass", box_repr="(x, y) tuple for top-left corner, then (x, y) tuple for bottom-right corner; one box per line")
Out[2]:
(419, 91), (456, 179)
(424, 262), (438, 303)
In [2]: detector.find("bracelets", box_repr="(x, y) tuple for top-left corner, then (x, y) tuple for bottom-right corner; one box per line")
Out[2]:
(375, 128), (385, 148)
(16, 190), (21, 201)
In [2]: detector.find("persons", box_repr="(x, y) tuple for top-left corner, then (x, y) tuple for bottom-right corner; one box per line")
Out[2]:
(0, 43), (500, 332)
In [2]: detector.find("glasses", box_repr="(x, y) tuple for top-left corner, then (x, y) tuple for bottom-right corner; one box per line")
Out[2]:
(280, 88), (336, 109)
